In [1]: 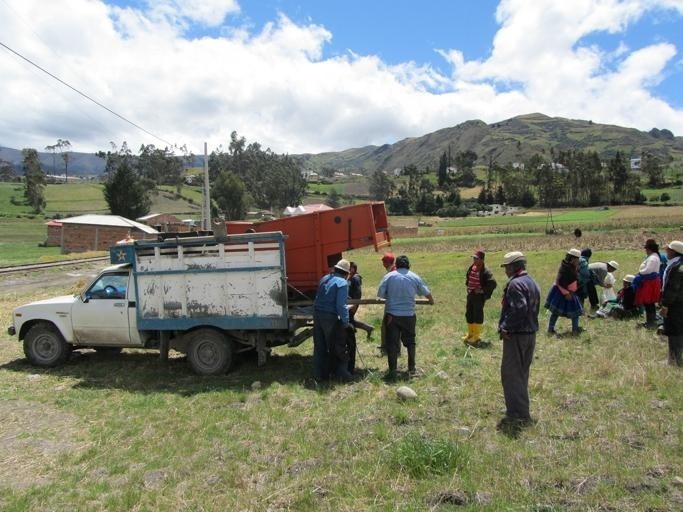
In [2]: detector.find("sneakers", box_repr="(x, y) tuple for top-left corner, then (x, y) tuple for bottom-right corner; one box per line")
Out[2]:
(381, 370), (415, 379)
(312, 370), (357, 381)
(364, 323), (375, 339)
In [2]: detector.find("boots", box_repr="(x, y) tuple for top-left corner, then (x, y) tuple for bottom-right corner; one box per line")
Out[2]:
(463, 323), (472, 341)
(468, 324), (480, 342)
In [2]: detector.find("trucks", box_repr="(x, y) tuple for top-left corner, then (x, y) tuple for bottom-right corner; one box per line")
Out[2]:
(7, 234), (357, 377)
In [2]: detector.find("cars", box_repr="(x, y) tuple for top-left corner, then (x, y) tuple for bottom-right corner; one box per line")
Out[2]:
(308, 172), (333, 185)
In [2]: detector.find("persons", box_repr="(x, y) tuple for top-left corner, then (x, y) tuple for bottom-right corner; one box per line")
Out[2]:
(545, 239), (683, 367)
(376, 255), (434, 374)
(461, 249), (497, 344)
(311, 258), (353, 382)
(348, 262), (374, 338)
(381, 252), (396, 273)
(499, 251), (540, 424)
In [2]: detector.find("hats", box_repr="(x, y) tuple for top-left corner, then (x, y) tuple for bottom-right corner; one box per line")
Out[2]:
(565, 248), (583, 258)
(334, 259), (351, 274)
(381, 253), (394, 264)
(644, 239), (659, 249)
(607, 260), (620, 270)
(667, 240), (683, 253)
(623, 274), (636, 283)
(470, 250), (486, 261)
(500, 251), (526, 267)
